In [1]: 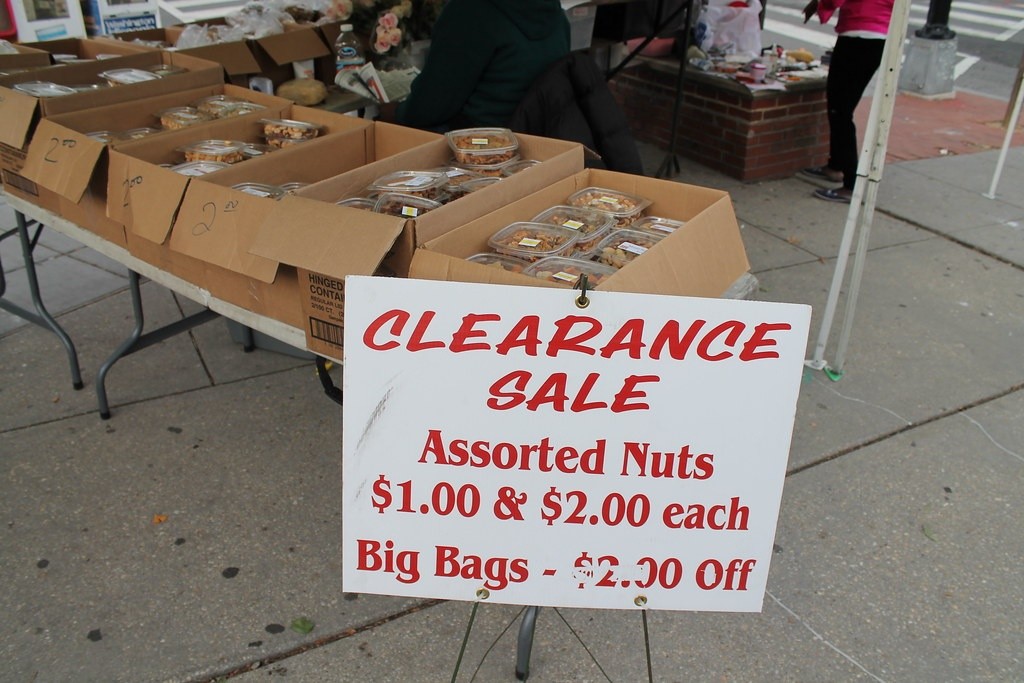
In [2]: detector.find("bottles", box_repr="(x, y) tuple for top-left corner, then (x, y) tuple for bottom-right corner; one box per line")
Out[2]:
(335, 24), (363, 58)
(768, 42), (778, 72)
(751, 63), (766, 79)
(336, 59), (364, 76)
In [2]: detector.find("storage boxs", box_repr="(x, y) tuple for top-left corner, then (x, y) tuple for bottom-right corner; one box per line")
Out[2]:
(0, 1), (755, 365)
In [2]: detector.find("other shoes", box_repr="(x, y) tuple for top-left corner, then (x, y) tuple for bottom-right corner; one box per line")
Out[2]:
(802, 165), (841, 182)
(815, 188), (852, 203)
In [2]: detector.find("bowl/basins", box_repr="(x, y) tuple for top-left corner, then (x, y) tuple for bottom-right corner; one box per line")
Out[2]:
(0, 40), (685, 291)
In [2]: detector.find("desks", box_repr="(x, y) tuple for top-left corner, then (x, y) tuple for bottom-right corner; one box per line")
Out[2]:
(0, 90), (760, 678)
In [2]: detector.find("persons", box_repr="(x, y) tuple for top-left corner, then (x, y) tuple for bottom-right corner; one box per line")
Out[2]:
(802, 0), (895, 203)
(374, 0), (572, 134)
